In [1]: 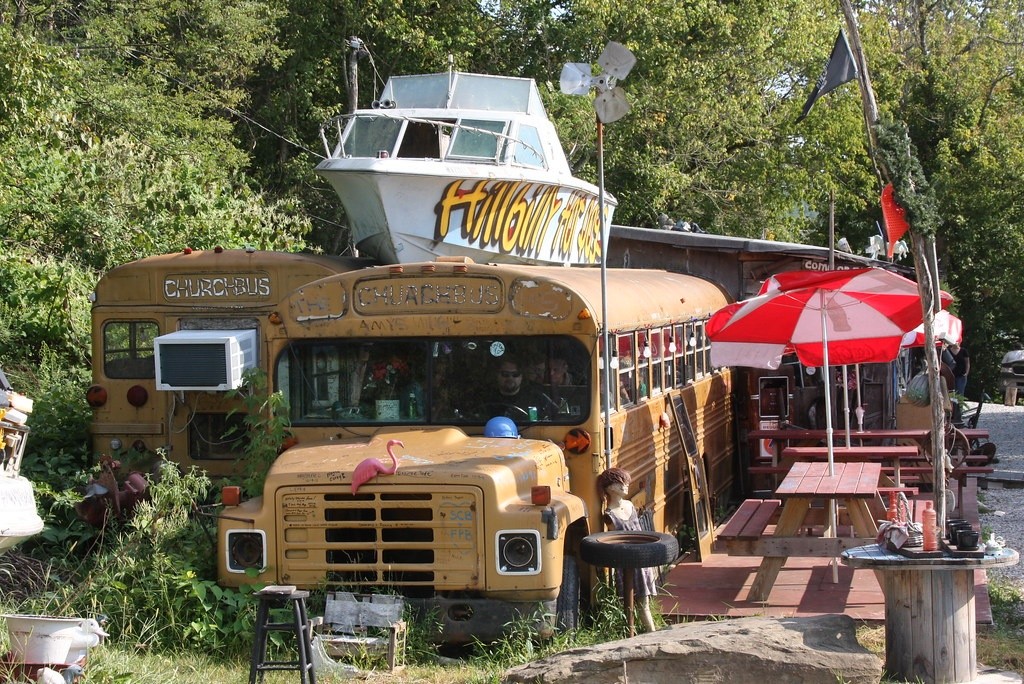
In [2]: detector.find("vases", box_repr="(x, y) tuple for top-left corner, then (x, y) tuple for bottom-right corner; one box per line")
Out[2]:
(946, 518), (979, 551)
(375, 400), (400, 423)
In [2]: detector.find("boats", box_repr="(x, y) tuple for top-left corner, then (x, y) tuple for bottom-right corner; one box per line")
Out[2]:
(313, 55), (620, 268)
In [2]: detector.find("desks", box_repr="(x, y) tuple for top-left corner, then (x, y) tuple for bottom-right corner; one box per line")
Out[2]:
(948, 428), (989, 440)
(747, 428), (930, 467)
(781, 445), (919, 487)
(770, 462), (889, 537)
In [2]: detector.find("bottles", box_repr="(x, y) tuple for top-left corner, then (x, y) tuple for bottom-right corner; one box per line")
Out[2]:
(408, 393), (418, 419)
(922, 501), (937, 551)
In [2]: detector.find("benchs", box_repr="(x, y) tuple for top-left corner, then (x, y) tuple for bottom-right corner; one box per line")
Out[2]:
(748, 455), (993, 498)
(710, 499), (788, 601)
(900, 498), (933, 523)
(308, 592), (407, 672)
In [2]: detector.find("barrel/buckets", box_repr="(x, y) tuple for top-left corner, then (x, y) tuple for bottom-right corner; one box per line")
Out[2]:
(0, 614), (83, 663)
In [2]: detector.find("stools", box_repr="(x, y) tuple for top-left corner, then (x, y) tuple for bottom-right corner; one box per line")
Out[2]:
(248, 591), (316, 684)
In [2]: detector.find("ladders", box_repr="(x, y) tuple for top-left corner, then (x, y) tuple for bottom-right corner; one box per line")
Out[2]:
(247, 587), (316, 684)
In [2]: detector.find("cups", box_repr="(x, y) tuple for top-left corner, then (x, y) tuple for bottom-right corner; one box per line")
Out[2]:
(528, 407), (538, 421)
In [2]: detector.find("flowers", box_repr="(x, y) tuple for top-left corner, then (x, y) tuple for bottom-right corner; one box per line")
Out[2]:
(363, 355), (409, 399)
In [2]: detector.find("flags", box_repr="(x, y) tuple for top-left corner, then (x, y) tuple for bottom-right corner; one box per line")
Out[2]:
(795, 28), (861, 124)
(880, 182), (910, 258)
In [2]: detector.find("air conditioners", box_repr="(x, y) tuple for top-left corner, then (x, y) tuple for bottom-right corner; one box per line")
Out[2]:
(154, 329), (258, 391)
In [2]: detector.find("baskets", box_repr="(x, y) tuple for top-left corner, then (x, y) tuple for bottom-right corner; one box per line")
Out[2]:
(887, 491), (940, 547)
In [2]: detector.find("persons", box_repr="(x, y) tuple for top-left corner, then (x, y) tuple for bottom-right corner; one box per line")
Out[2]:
(945, 341), (971, 397)
(474, 357), (549, 422)
(598, 468), (658, 634)
(600, 356), (698, 410)
(542, 355), (579, 394)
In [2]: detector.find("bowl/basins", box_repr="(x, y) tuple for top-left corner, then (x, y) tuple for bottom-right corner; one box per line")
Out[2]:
(949, 523), (961, 540)
(946, 519), (967, 536)
(957, 530), (979, 547)
(953, 524), (972, 538)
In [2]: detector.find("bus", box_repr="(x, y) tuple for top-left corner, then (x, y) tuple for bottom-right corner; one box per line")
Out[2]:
(85, 237), (735, 652)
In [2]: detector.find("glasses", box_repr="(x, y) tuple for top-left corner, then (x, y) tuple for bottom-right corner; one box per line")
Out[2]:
(501, 373), (520, 377)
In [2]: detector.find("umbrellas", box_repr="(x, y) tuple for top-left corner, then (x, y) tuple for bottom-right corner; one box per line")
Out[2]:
(706, 267), (963, 584)
